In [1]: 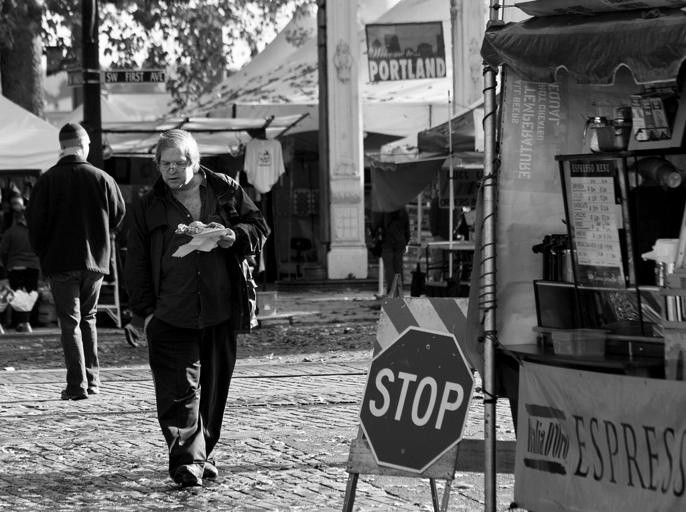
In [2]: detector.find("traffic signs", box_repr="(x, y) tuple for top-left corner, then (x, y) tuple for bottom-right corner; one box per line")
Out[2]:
(103, 69), (166, 83)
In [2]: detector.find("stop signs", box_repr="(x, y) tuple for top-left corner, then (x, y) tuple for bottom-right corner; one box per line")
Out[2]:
(358, 326), (475, 475)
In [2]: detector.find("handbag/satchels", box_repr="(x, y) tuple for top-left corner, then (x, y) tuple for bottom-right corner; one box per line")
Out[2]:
(239, 256), (258, 328)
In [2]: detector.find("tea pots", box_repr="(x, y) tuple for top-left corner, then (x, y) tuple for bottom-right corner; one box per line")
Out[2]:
(583, 117), (615, 153)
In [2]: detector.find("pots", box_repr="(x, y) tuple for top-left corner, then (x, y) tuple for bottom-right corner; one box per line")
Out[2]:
(532, 233), (577, 284)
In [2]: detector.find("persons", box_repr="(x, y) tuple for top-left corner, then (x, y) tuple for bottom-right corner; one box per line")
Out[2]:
(25, 123), (126, 401)
(370, 205), (411, 296)
(1, 195), (42, 332)
(123, 129), (270, 484)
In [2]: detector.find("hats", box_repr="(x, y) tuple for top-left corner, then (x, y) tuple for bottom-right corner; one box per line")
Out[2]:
(59, 122), (91, 148)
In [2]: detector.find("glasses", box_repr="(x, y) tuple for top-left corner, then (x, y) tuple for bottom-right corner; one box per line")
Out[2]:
(157, 163), (186, 173)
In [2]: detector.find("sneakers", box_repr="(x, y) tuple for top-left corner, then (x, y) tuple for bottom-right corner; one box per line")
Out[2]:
(61, 390), (87, 400)
(174, 463), (203, 487)
(203, 461), (218, 479)
(87, 385), (100, 394)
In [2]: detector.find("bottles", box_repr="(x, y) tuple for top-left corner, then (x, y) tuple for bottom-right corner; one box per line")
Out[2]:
(628, 156), (682, 189)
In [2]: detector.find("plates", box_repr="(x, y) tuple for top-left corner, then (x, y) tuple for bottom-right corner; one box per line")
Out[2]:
(193, 229), (228, 239)
